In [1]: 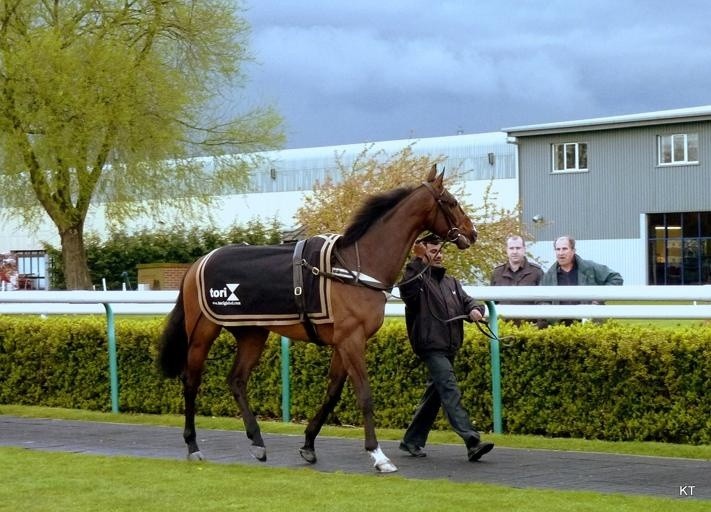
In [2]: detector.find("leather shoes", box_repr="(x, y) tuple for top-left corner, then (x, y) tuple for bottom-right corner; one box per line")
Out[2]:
(398, 439), (426, 457)
(467, 443), (493, 461)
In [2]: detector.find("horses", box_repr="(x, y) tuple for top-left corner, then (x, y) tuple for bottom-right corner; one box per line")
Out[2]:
(158, 163), (479, 473)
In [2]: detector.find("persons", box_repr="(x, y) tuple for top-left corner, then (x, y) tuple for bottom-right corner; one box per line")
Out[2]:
(535, 236), (623, 329)
(398, 234), (494, 464)
(486, 237), (545, 328)
